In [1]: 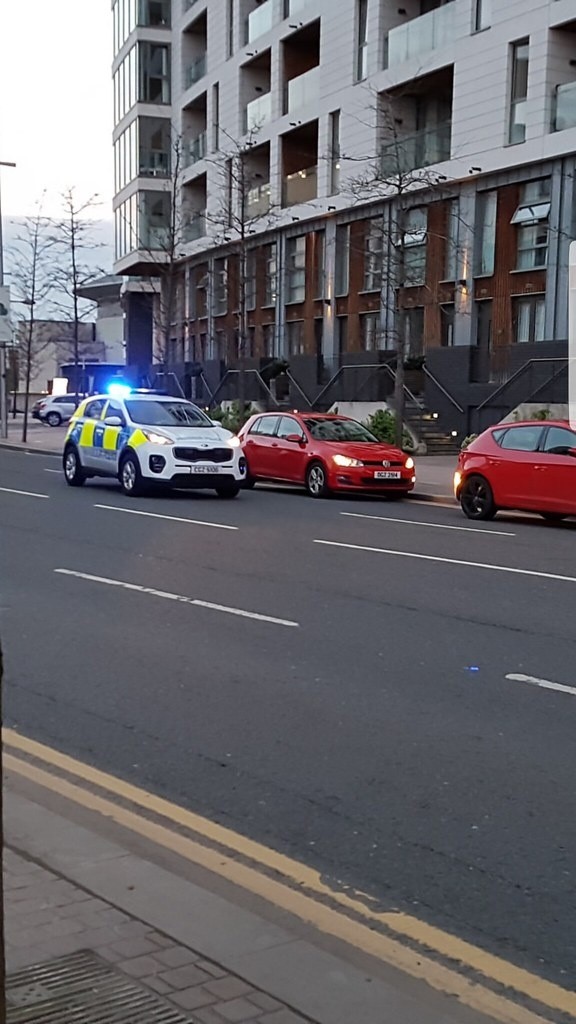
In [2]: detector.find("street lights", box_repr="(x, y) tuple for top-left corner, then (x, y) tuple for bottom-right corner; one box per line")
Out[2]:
(0, 162), (16, 439)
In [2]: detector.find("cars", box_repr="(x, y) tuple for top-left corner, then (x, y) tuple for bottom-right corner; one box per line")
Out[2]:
(236, 409), (416, 502)
(30, 389), (99, 427)
(62, 385), (247, 499)
(454, 420), (576, 522)
